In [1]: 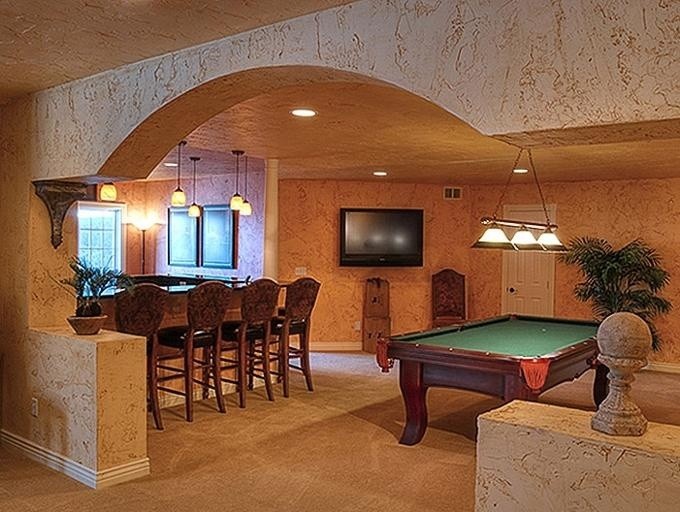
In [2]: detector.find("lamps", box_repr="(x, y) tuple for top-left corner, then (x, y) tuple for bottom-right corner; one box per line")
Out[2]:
(172, 140), (201, 217)
(471, 147), (569, 254)
(230, 149), (252, 216)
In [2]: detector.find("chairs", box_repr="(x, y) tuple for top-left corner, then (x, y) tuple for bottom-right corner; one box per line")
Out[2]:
(114, 276), (321, 430)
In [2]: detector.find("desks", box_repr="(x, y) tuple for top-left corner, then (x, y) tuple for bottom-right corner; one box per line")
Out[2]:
(382, 314), (603, 446)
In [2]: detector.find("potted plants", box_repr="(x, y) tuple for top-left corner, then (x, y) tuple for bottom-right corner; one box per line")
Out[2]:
(61, 254), (135, 336)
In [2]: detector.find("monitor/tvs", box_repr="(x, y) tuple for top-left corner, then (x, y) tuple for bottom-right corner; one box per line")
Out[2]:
(338, 207), (425, 268)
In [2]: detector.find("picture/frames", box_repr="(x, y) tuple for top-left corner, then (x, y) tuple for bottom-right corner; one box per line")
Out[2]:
(166, 204), (236, 270)
